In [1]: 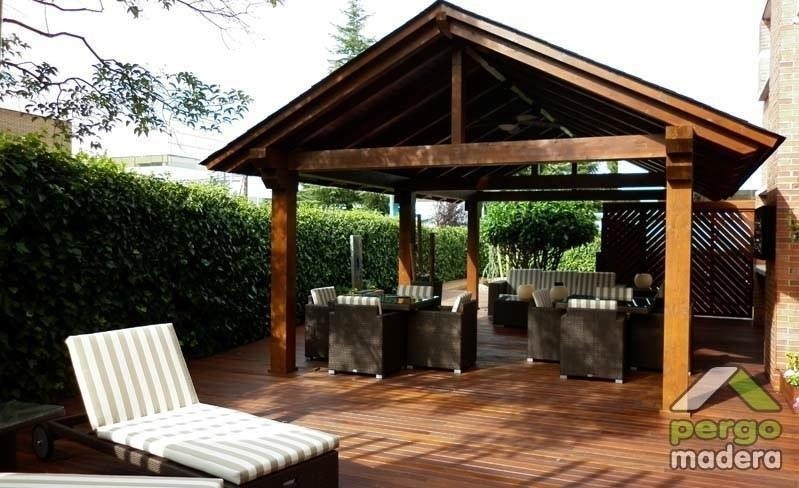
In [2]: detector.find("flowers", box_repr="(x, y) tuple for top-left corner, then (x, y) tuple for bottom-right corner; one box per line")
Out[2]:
(782, 351), (799, 390)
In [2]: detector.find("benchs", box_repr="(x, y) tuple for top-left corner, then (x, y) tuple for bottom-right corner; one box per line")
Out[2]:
(0, 470), (225, 487)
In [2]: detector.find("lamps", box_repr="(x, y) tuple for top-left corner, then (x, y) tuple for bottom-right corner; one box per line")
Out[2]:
(495, 80), (522, 132)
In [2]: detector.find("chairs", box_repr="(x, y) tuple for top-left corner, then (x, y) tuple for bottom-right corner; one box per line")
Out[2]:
(28, 319), (343, 487)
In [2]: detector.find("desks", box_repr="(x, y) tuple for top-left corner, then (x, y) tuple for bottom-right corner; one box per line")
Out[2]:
(0, 398), (67, 472)
